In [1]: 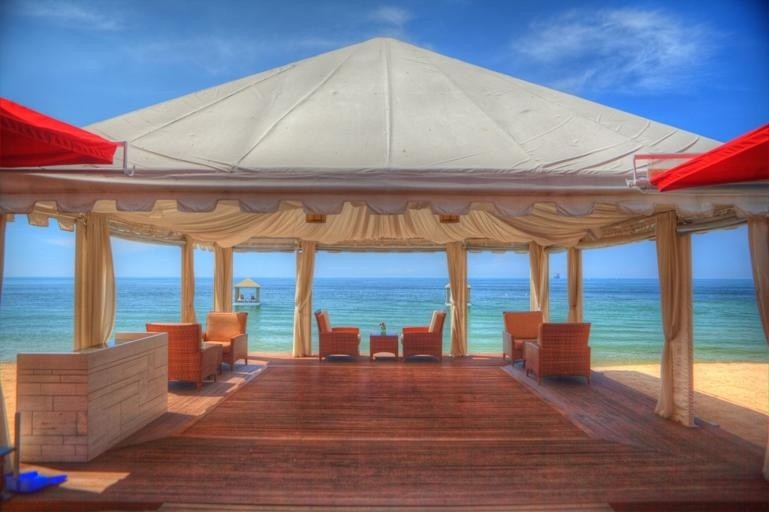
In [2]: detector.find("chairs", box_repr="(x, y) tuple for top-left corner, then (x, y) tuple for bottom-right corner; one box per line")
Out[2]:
(504, 311), (592, 386)
(400, 310), (448, 362)
(314, 309), (360, 361)
(145, 312), (249, 392)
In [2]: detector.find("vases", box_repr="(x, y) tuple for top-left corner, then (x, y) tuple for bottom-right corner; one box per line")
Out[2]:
(381, 328), (386, 335)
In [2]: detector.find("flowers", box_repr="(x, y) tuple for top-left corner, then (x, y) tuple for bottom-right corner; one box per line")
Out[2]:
(379, 321), (385, 328)
